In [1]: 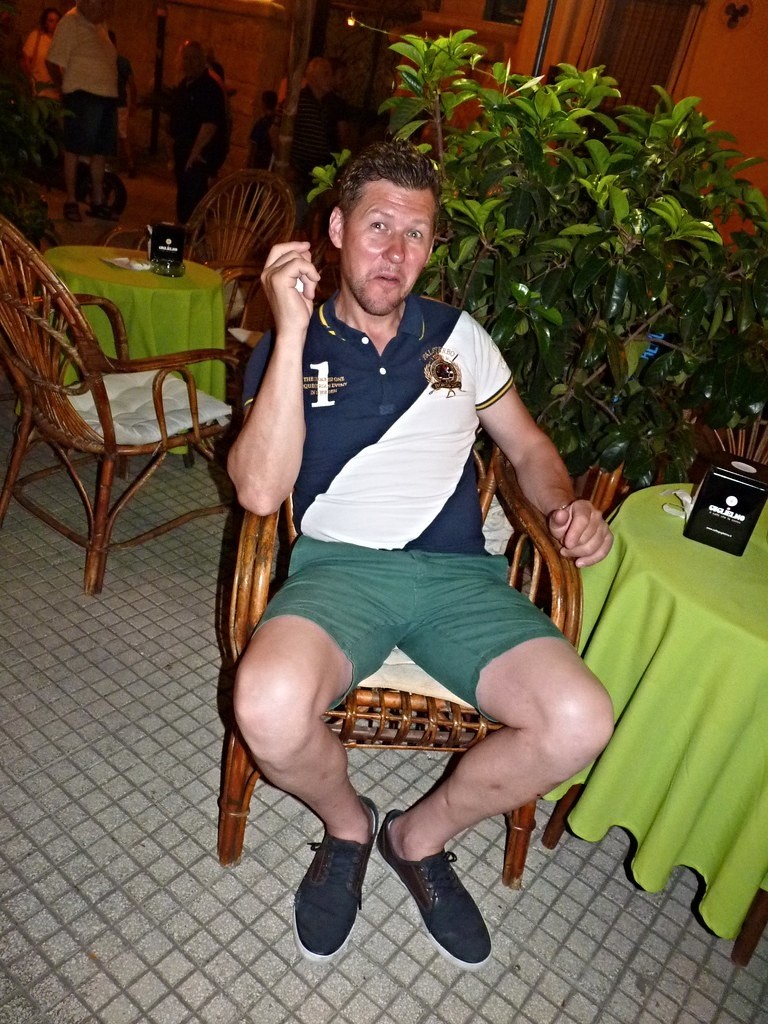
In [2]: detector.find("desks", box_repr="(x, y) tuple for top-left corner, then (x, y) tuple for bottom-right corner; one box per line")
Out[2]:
(14, 246), (224, 454)
(540, 482), (768, 966)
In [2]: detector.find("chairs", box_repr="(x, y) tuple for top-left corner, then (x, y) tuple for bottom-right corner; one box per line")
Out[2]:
(101, 171), (298, 402)
(217, 427), (582, 890)
(0, 214), (243, 593)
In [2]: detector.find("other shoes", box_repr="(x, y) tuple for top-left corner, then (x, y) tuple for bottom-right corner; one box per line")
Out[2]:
(90, 201), (119, 221)
(63, 202), (82, 224)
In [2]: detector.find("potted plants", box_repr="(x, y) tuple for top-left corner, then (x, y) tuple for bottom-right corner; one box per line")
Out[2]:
(319, 29), (741, 557)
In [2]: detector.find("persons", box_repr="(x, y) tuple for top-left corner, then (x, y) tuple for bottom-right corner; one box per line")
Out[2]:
(107, 30), (136, 180)
(45, 0), (119, 222)
(243, 91), (278, 221)
(271, 57), (388, 241)
(22, 6), (62, 102)
(167, 40), (231, 223)
(225, 141), (614, 971)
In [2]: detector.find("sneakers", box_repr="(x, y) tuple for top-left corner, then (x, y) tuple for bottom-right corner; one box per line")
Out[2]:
(294, 796), (378, 962)
(375, 809), (493, 969)
